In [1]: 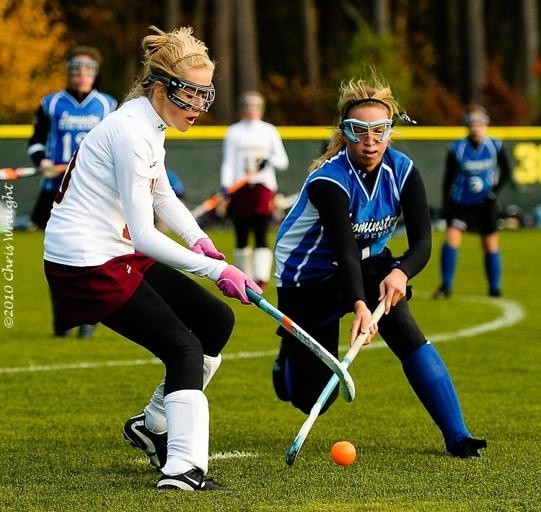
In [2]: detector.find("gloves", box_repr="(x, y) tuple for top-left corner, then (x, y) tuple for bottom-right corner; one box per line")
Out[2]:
(190, 238), (225, 260)
(215, 264), (264, 305)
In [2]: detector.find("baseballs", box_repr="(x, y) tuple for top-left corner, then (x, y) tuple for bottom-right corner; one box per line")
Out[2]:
(331, 441), (356, 466)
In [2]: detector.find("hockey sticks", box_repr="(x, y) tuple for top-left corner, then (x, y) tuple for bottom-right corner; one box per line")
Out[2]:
(282, 294), (387, 466)
(245, 286), (356, 403)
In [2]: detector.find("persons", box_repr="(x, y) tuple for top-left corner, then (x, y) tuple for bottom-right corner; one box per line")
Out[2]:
(430, 103), (512, 300)
(271, 62), (490, 459)
(41, 23), (265, 492)
(216, 90), (291, 289)
(0, 139), (540, 240)
(25, 44), (121, 340)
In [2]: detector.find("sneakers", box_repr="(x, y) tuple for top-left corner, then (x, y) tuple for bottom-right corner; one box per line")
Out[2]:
(156, 465), (229, 491)
(122, 411), (168, 477)
